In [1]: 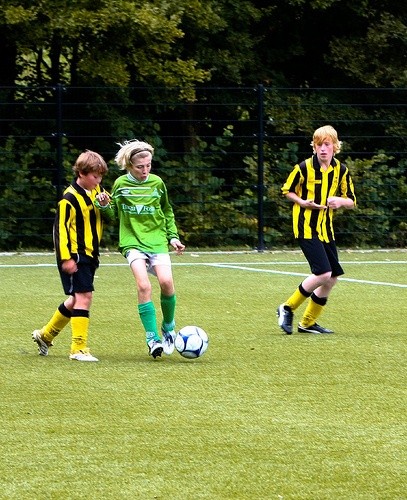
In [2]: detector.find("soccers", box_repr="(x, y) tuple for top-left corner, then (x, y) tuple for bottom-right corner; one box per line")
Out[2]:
(174, 325), (209, 359)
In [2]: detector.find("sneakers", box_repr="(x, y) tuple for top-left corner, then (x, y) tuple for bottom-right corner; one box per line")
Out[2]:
(31, 329), (48, 356)
(161, 324), (174, 355)
(297, 322), (334, 335)
(276, 303), (293, 335)
(68, 348), (99, 362)
(148, 342), (163, 359)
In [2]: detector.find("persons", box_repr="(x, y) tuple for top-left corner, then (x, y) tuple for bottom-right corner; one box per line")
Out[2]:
(276, 125), (356, 334)
(93, 141), (186, 358)
(31, 149), (111, 362)
